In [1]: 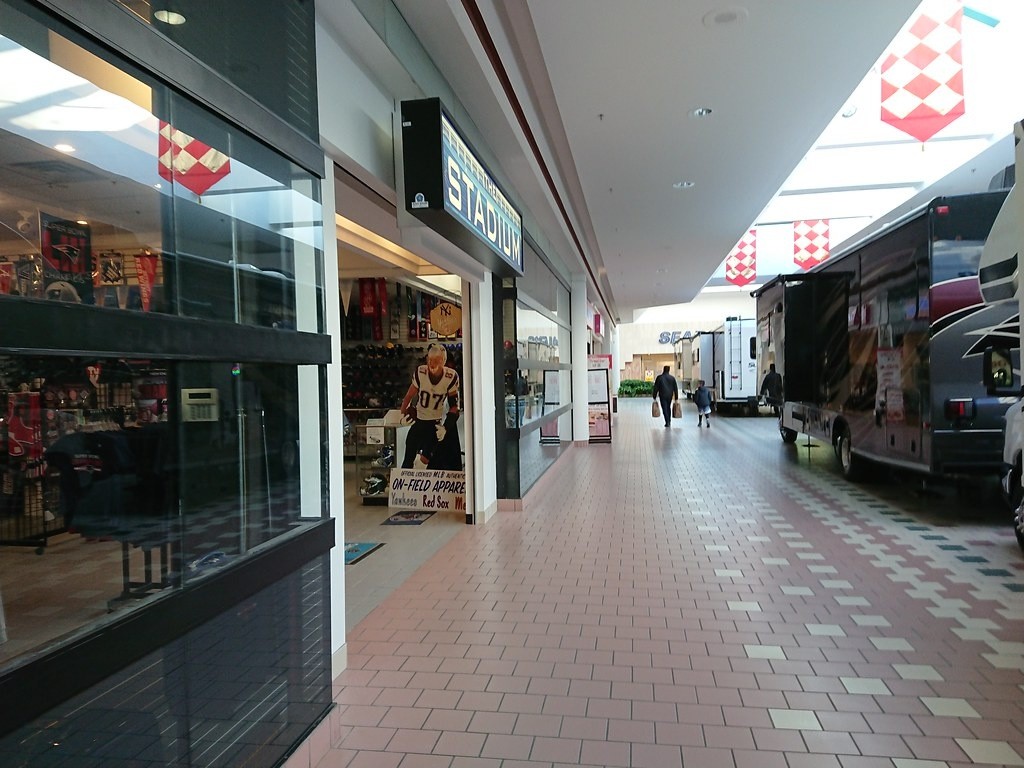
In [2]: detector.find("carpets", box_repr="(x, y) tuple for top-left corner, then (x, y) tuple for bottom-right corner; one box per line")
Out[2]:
(345, 543), (386, 565)
(380, 511), (437, 525)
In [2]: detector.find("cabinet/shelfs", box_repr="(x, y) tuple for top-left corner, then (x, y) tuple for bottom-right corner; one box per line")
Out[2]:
(355, 422), (412, 506)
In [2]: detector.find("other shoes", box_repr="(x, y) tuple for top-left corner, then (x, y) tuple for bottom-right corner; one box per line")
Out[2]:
(664, 422), (671, 427)
(707, 423), (710, 428)
(698, 423), (702, 427)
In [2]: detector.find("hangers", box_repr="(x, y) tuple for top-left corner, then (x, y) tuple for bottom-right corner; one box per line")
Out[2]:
(78, 407), (142, 434)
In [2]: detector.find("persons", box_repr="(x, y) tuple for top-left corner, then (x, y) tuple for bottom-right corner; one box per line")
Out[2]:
(694, 381), (711, 429)
(759, 364), (783, 404)
(652, 366), (679, 428)
(399, 346), (461, 469)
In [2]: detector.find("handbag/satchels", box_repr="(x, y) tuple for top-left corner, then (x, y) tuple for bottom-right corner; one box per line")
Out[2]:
(672, 400), (683, 418)
(652, 399), (660, 417)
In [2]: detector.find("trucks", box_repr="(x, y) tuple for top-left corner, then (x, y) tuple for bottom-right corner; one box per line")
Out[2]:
(749, 123), (1024, 483)
(673, 315), (765, 418)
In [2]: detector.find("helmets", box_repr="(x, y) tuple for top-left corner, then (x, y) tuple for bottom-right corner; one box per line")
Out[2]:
(359, 473), (387, 496)
(370, 443), (396, 469)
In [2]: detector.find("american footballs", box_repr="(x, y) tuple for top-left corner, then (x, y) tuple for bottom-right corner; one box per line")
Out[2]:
(404, 406), (418, 424)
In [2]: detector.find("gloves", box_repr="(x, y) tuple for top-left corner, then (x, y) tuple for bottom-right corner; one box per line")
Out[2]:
(435, 424), (447, 442)
(399, 414), (416, 427)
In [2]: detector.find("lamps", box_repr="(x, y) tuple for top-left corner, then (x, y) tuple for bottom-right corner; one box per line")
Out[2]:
(15, 209), (33, 233)
(154, 0), (186, 25)
